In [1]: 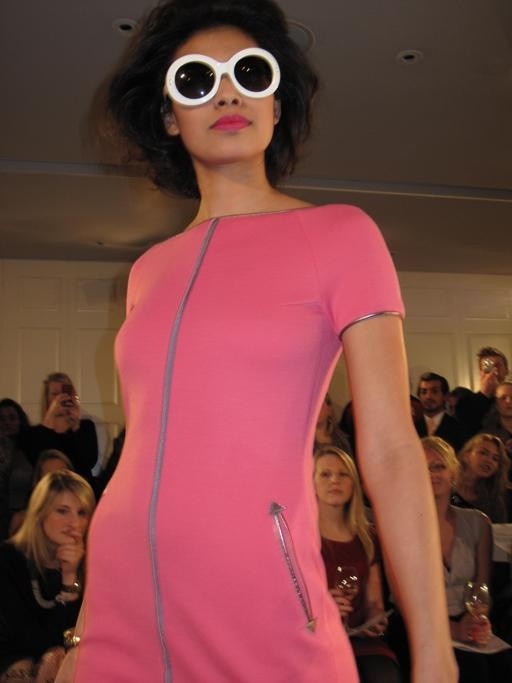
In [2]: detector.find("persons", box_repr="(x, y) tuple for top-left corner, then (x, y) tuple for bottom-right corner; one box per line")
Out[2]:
(60, 9), (463, 679)
(402, 347), (512, 683)
(312, 390), (417, 682)
(1, 375), (96, 682)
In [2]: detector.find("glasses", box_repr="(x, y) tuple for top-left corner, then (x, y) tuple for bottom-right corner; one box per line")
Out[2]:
(160, 45), (284, 108)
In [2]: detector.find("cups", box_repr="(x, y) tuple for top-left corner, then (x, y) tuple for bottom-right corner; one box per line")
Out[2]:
(461, 582), (492, 619)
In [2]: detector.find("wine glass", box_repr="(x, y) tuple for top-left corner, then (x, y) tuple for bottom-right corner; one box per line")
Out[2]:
(335, 567), (360, 625)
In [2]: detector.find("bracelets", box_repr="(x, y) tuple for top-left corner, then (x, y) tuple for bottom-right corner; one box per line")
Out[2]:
(63, 635), (81, 649)
